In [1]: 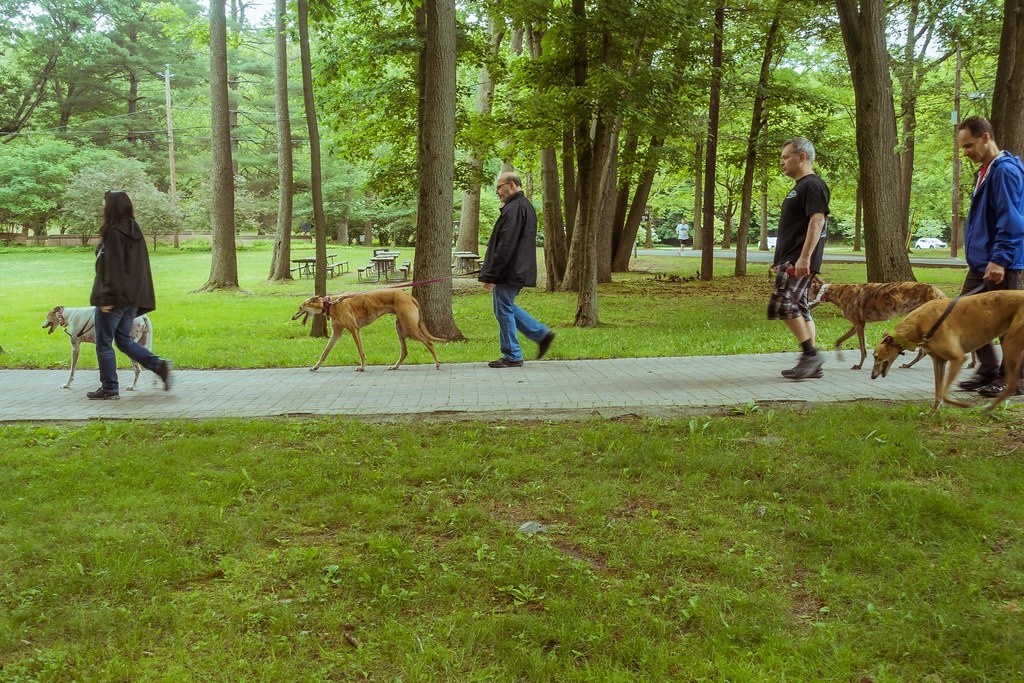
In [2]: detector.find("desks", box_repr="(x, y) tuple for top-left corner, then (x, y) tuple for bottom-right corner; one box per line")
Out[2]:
(453, 251), (482, 273)
(292, 253), (338, 278)
(370, 252), (400, 284)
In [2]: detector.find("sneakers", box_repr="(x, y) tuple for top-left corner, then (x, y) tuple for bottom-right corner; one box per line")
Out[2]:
(489, 357), (526, 368)
(978, 374), (1024, 398)
(85, 387), (120, 400)
(537, 331), (557, 360)
(959, 367), (1001, 390)
(781, 351), (826, 380)
(160, 358), (175, 392)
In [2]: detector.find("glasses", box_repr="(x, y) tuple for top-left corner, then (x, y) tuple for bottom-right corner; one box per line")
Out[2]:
(496, 181), (512, 191)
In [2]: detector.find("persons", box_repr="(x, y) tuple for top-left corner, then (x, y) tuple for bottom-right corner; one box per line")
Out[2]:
(958, 116), (1024, 397)
(477, 171), (555, 368)
(766, 137), (830, 379)
(675, 219), (689, 252)
(85, 190), (173, 399)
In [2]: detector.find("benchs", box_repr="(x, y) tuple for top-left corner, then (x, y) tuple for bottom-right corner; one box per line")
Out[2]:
(479, 262), (484, 267)
(474, 259), (483, 267)
(289, 261), (350, 280)
(356, 261), (378, 284)
(394, 259), (412, 283)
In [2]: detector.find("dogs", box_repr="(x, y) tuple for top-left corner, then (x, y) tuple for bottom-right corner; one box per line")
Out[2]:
(292, 288), (446, 371)
(871, 290), (1024, 419)
(42, 305), (152, 392)
(808, 282), (948, 370)
